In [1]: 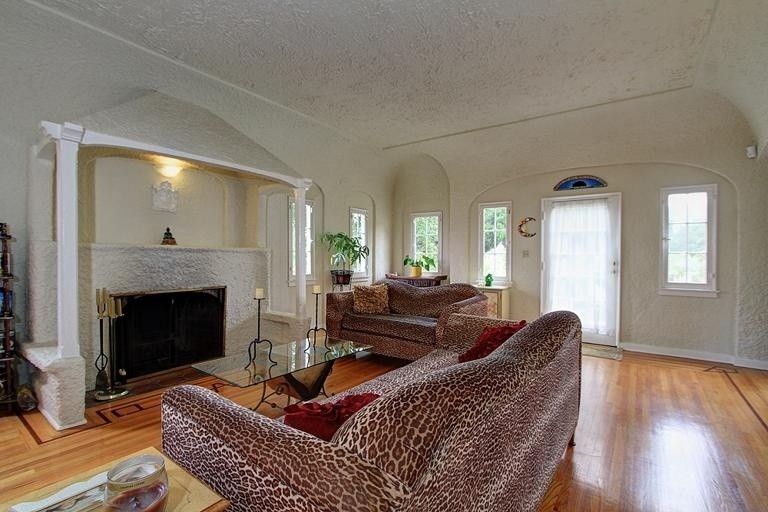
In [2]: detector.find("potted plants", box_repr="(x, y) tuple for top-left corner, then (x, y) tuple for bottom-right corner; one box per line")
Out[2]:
(404, 254), (435, 277)
(320, 231), (369, 285)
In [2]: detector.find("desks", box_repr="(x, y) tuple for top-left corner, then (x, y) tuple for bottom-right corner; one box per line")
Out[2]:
(0, 445), (231, 512)
(385, 272), (447, 287)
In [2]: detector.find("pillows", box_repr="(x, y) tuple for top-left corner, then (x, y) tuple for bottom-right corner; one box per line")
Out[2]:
(353, 284), (389, 315)
(458, 320), (527, 362)
(285, 393), (380, 441)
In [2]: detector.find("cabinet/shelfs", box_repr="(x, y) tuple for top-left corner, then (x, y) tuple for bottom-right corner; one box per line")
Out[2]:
(0, 222), (17, 411)
(474, 285), (510, 320)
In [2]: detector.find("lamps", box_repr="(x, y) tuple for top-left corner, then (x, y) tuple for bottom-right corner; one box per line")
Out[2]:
(152, 162), (184, 178)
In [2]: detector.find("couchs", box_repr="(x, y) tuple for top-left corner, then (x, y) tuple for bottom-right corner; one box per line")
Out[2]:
(326, 279), (488, 362)
(161, 310), (582, 511)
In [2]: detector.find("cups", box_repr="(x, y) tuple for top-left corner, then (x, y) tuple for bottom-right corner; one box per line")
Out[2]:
(102, 454), (169, 512)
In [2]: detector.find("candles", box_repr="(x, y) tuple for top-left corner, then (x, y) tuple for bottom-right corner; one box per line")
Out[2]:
(313, 286), (320, 293)
(96, 289), (101, 305)
(256, 288), (263, 299)
(116, 298), (122, 314)
(102, 287), (107, 303)
(109, 299), (115, 315)
